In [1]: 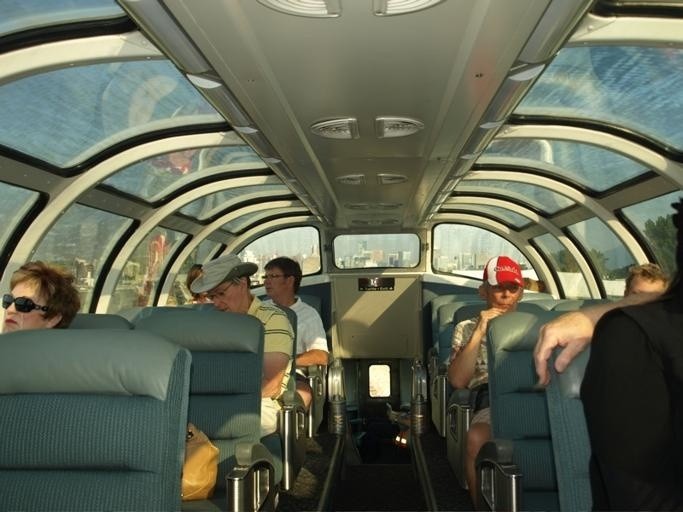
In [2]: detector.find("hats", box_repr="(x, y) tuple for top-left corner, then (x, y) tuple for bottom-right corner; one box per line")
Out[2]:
(484, 256), (523, 288)
(190, 252), (257, 293)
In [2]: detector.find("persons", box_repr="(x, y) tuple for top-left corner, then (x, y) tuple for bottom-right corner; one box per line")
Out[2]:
(261, 257), (329, 412)
(580, 197), (683, 512)
(447, 256), (524, 512)
(2, 260), (80, 332)
(533, 293), (663, 386)
(184, 265), (214, 304)
(190, 253), (295, 440)
(623, 262), (671, 295)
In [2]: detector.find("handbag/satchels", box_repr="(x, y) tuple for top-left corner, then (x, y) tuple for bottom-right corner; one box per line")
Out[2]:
(180, 423), (220, 501)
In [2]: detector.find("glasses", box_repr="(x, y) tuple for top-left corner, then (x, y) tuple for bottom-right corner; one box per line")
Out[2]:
(264, 273), (284, 281)
(207, 280), (234, 300)
(2, 294), (49, 312)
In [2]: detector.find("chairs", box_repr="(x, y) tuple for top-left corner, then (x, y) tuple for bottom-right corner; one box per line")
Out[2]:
(0, 294), (326, 512)
(430, 289), (615, 511)
